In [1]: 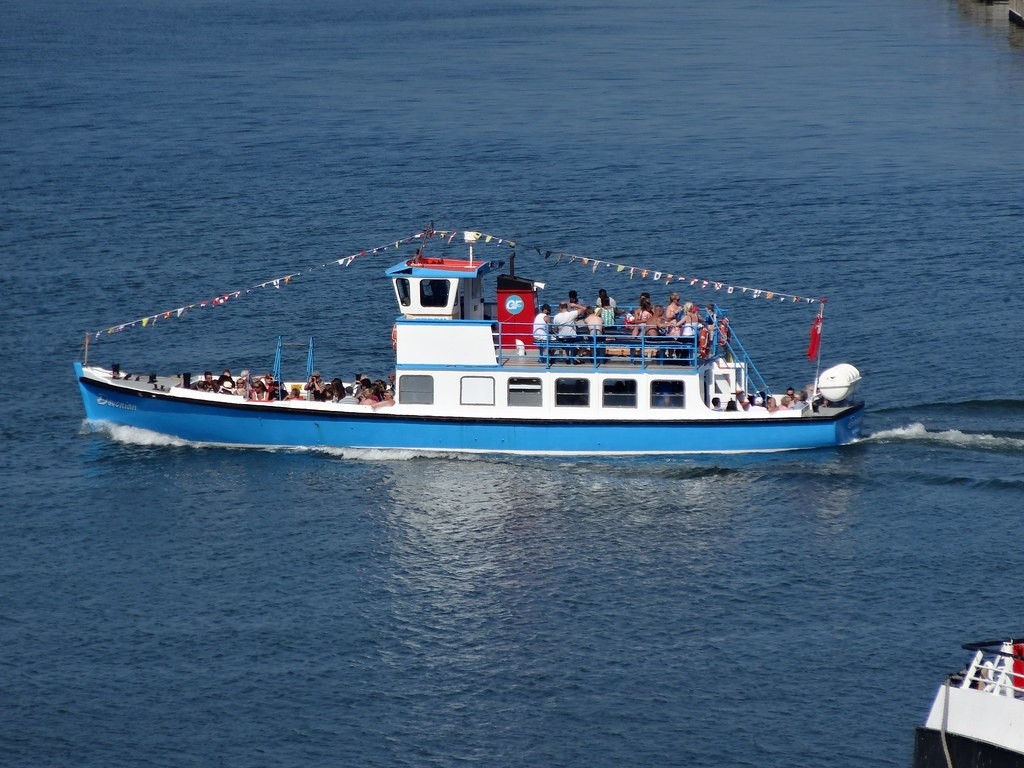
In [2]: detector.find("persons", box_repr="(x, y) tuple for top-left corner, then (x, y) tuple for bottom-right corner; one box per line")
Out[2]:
(192, 369), (306, 401)
(304, 371), (396, 409)
(710, 398), (723, 411)
(112, 363), (120, 379)
(625, 293), (734, 365)
(726, 401), (737, 410)
(767, 388), (808, 412)
(533, 289), (626, 364)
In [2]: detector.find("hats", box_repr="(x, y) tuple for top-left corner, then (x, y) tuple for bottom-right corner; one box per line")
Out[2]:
(693, 306), (699, 312)
(312, 371), (320, 376)
(241, 370), (251, 377)
(706, 304), (714, 310)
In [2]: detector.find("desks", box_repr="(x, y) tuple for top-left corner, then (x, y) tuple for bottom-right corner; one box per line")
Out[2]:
(551, 316), (666, 336)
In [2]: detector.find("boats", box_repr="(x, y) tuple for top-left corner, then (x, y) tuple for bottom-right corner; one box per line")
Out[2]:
(914, 637), (1023, 768)
(72, 222), (870, 459)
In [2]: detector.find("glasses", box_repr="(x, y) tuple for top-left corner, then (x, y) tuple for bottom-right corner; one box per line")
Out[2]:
(267, 377), (273, 380)
(675, 299), (680, 302)
(237, 382), (243, 384)
(787, 394), (793, 396)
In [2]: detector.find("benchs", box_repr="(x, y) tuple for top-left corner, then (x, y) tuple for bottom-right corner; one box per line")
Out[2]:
(534, 340), (694, 366)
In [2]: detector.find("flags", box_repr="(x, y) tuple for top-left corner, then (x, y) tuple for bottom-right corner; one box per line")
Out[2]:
(81, 233), (425, 348)
(273, 339), (281, 377)
(306, 341), (313, 377)
(428, 220), (434, 238)
(807, 310), (823, 360)
(439, 230), (825, 304)
